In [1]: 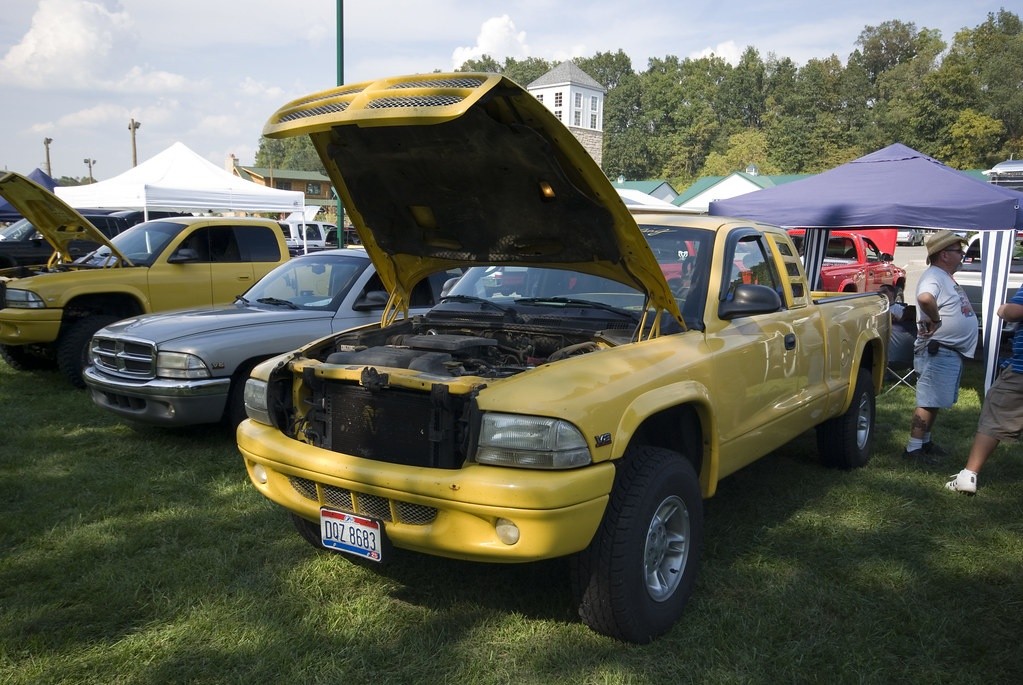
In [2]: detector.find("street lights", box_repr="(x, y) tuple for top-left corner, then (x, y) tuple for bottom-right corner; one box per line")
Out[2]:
(128, 118), (141, 169)
(84, 158), (96, 184)
(44, 137), (55, 177)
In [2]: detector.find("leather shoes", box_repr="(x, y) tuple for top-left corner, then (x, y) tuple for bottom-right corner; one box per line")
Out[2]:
(902, 447), (938, 464)
(923, 440), (950, 456)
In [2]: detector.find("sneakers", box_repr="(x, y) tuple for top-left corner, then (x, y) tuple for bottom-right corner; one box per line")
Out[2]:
(945, 470), (977, 493)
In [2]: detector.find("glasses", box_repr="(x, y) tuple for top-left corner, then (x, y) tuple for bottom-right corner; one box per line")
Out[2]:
(946, 246), (963, 253)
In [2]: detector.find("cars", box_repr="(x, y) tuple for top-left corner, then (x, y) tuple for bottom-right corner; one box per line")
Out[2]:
(896, 229), (927, 247)
(107, 211), (195, 226)
(1, 211), (133, 271)
(323, 227), (364, 252)
(496, 229), (757, 300)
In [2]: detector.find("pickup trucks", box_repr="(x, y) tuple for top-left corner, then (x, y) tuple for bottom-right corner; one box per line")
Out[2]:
(234, 72), (892, 646)
(0, 170), (333, 394)
(785, 229), (907, 304)
(83, 250), (523, 437)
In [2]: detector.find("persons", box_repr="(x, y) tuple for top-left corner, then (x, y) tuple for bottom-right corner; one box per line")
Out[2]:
(902, 230), (979, 466)
(879, 301), (918, 386)
(944, 284), (1023, 493)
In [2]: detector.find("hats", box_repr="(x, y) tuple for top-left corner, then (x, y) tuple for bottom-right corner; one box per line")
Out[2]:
(925, 230), (968, 264)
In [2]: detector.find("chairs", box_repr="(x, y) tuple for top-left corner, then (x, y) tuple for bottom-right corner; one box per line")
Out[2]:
(885, 305), (916, 394)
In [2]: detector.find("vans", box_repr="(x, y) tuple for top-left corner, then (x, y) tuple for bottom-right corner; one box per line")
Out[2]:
(278, 221), (340, 259)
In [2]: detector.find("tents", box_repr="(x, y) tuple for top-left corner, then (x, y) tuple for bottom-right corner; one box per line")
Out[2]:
(708, 142), (1023, 398)
(0, 141), (308, 256)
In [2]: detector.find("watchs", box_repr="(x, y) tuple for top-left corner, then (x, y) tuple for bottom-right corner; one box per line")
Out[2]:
(931, 316), (942, 323)
